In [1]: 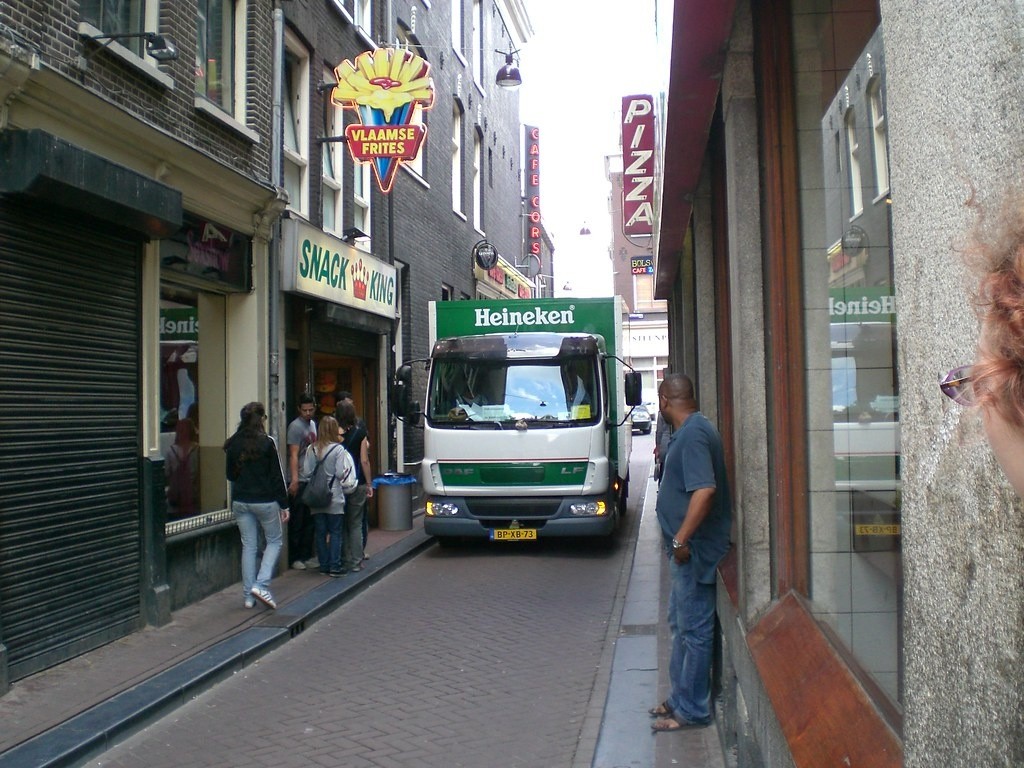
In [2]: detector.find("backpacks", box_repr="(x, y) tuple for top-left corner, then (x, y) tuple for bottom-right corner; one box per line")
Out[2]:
(168, 442), (199, 502)
(301, 443), (340, 508)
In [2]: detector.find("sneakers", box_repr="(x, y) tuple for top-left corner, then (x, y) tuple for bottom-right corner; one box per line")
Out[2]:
(251, 587), (277, 609)
(244, 598), (257, 608)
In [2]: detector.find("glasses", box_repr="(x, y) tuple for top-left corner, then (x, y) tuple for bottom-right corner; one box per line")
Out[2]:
(939, 364), (1024, 406)
(265, 415), (267, 420)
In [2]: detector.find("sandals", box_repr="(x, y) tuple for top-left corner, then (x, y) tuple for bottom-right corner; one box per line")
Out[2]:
(648, 702), (674, 717)
(650, 714), (709, 731)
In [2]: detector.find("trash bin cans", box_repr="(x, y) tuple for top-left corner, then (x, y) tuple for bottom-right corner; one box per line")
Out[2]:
(372, 472), (412, 532)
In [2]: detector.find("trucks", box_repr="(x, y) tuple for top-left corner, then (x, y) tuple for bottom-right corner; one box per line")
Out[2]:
(389, 298), (642, 549)
(825, 287), (902, 555)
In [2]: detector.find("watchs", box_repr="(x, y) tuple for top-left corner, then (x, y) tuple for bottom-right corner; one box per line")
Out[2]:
(673, 539), (686, 549)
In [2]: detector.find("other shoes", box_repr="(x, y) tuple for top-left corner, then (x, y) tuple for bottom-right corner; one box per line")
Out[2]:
(330, 570), (347, 577)
(292, 560), (306, 570)
(320, 570), (330, 575)
(364, 553), (371, 559)
(352, 564), (361, 571)
(305, 559), (320, 569)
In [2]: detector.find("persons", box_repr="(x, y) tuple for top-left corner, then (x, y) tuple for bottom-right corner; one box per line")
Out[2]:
(648, 374), (732, 732)
(165, 417), (199, 518)
(333, 391), (373, 572)
(453, 377), (489, 407)
(304, 416), (349, 577)
(655, 411), (673, 495)
(286, 396), (318, 569)
(223, 402), (290, 611)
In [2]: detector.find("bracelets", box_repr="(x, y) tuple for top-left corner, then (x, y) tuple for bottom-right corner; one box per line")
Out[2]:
(281, 508), (289, 511)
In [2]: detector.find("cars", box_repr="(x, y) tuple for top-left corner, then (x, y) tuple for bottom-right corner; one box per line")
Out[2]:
(630, 404), (652, 434)
